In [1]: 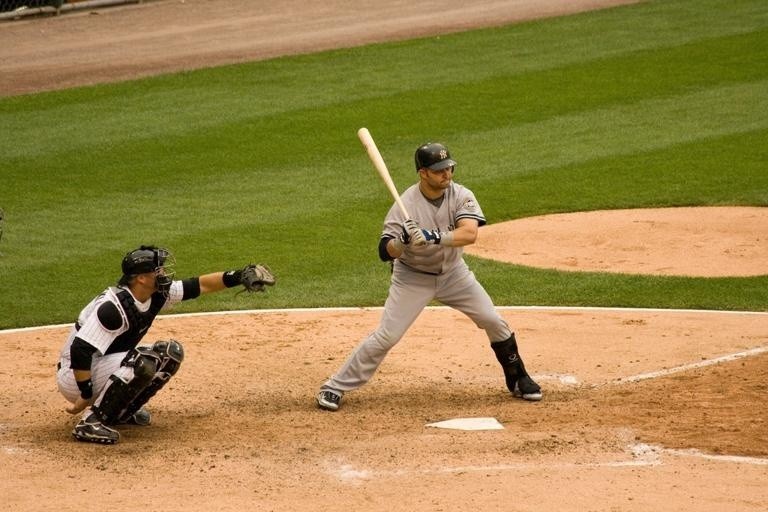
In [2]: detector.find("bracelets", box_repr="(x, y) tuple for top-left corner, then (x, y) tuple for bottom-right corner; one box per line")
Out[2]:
(223, 269), (243, 288)
(433, 229), (455, 248)
(392, 233), (409, 253)
(76, 377), (94, 401)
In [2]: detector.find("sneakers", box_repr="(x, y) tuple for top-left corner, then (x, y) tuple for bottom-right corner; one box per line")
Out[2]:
(513, 385), (542, 401)
(126, 409), (152, 426)
(318, 391), (341, 410)
(71, 419), (120, 444)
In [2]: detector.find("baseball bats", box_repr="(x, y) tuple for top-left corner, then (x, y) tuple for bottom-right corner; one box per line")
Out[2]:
(357, 127), (425, 247)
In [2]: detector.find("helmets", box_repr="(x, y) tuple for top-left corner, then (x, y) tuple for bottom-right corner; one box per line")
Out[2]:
(415, 142), (457, 171)
(119, 244), (176, 302)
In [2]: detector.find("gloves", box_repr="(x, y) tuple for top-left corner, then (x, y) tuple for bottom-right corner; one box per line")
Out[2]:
(409, 229), (441, 247)
(400, 219), (419, 245)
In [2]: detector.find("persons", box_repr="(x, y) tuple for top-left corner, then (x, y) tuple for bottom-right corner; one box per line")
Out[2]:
(315, 141), (542, 411)
(57, 244), (275, 445)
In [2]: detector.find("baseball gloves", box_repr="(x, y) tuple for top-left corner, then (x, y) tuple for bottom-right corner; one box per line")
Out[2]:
(234, 263), (275, 301)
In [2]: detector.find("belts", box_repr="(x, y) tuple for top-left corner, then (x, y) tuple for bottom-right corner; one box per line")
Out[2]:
(57, 362), (61, 370)
